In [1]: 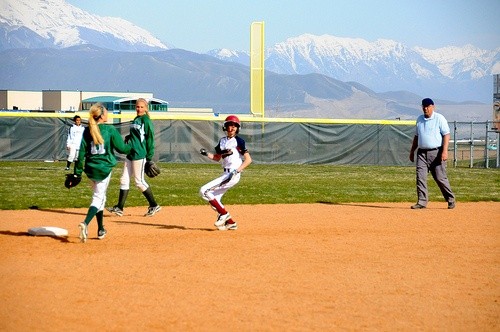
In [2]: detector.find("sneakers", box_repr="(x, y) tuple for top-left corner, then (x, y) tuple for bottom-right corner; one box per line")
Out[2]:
(214, 211), (231, 227)
(217, 221), (238, 231)
(144, 204), (161, 217)
(106, 205), (124, 217)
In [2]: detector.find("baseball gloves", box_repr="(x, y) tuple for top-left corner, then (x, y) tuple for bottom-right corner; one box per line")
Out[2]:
(64, 173), (82, 190)
(144, 161), (162, 178)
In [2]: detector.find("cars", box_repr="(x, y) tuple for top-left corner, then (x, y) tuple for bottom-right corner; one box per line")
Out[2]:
(489, 144), (497, 150)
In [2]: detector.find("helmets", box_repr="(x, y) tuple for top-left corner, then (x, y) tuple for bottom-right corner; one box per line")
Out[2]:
(222, 115), (241, 133)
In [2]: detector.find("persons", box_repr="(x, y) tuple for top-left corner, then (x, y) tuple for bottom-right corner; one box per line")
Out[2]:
(65, 116), (85, 171)
(106, 98), (161, 217)
(409, 99), (456, 209)
(74, 103), (132, 242)
(199, 116), (252, 230)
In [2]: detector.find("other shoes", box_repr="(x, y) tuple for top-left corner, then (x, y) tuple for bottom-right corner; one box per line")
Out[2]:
(411, 204), (426, 209)
(97, 229), (107, 239)
(447, 202), (456, 209)
(79, 222), (88, 243)
